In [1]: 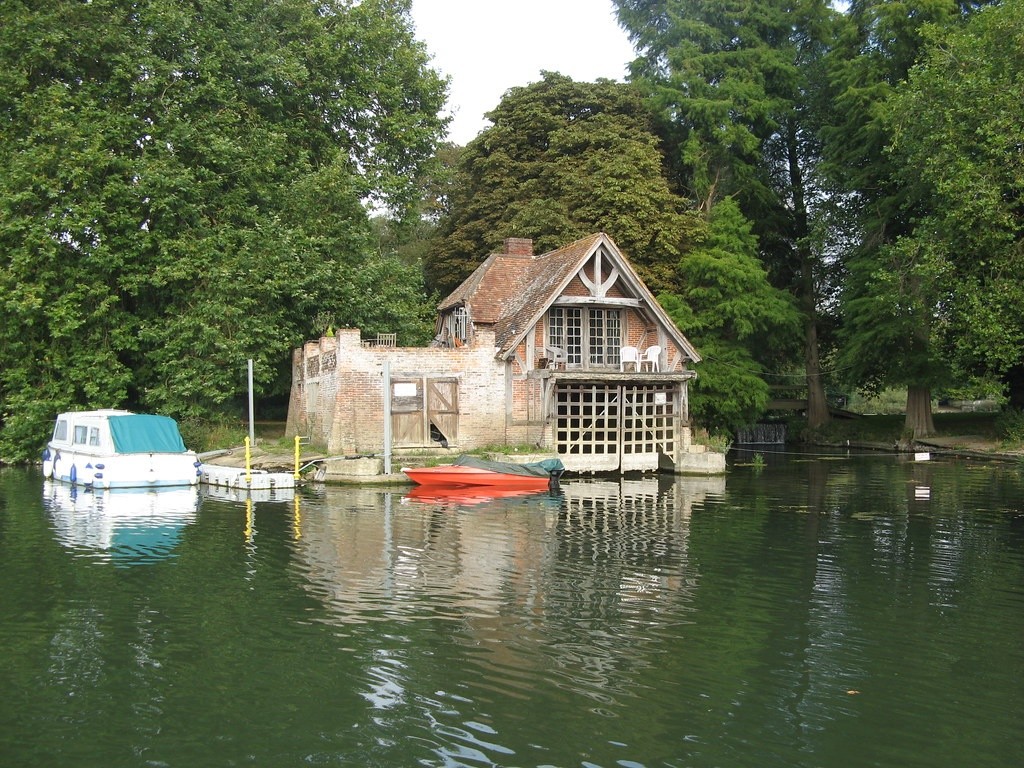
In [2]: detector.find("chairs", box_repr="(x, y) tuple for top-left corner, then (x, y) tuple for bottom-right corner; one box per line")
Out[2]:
(545, 347), (568, 369)
(638, 346), (662, 373)
(620, 346), (639, 373)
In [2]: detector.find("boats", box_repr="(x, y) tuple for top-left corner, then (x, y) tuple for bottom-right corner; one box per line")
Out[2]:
(404, 456), (563, 492)
(42, 409), (202, 489)
